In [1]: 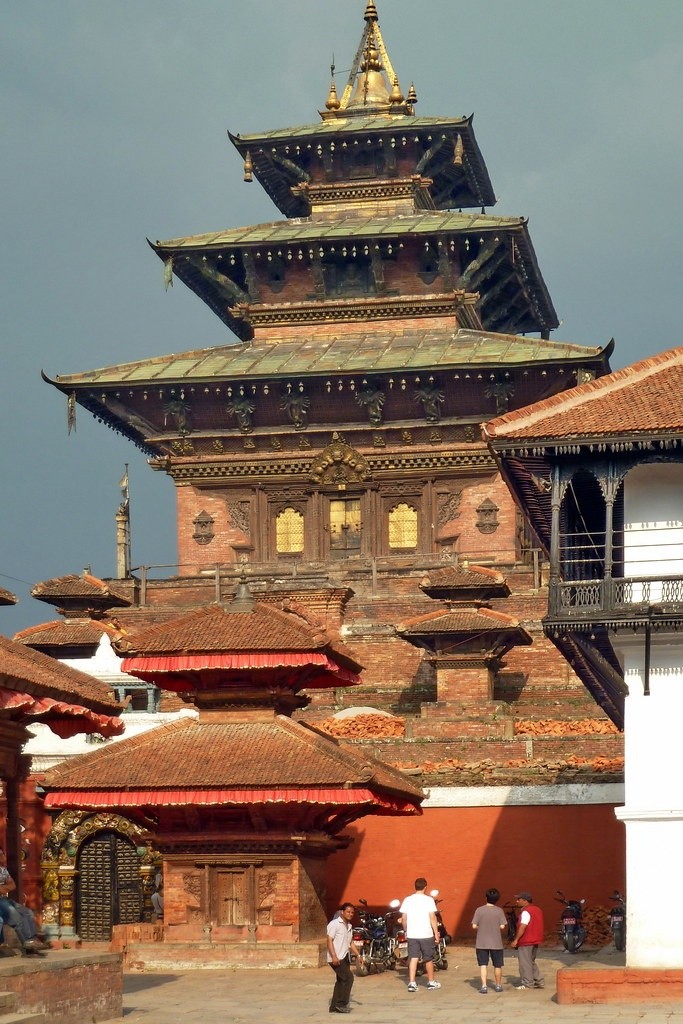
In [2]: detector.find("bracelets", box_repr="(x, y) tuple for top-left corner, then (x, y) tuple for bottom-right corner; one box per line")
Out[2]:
(356, 954), (361, 957)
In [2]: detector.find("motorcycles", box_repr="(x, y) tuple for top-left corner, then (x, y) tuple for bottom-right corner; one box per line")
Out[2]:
(349, 899), (451, 978)
(608, 890), (626, 951)
(554, 890), (588, 953)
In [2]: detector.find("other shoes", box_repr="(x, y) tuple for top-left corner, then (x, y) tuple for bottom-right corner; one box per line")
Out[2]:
(532, 983), (545, 988)
(0, 946), (15, 956)
(21, 948), (45, 958)
(480, 985), (488, 993)
(23, 937), (46, 949)
(516, 984), (528, 989)
(495, 984), (503, 992)
(330, 1006), (351, 1013)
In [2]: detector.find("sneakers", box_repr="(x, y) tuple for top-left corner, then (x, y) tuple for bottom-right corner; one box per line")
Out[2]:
(408, 981), (419, 992)
(427, 980), (441, 990)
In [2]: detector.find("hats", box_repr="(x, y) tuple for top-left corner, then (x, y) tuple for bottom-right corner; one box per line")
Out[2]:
(514, 892), (532, 903)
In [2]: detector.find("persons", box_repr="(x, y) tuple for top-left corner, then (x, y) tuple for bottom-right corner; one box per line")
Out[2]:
(511, 892), (545, 990)
(0, 846), (49, 949)
(326, 902), (364, 1013)
(151, 885), (164, 920)
(471, 888), (507, 993)
(399, 878), (441, 992)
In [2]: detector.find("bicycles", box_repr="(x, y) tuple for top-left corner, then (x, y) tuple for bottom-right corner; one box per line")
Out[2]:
(504, 902), (523, 951)
